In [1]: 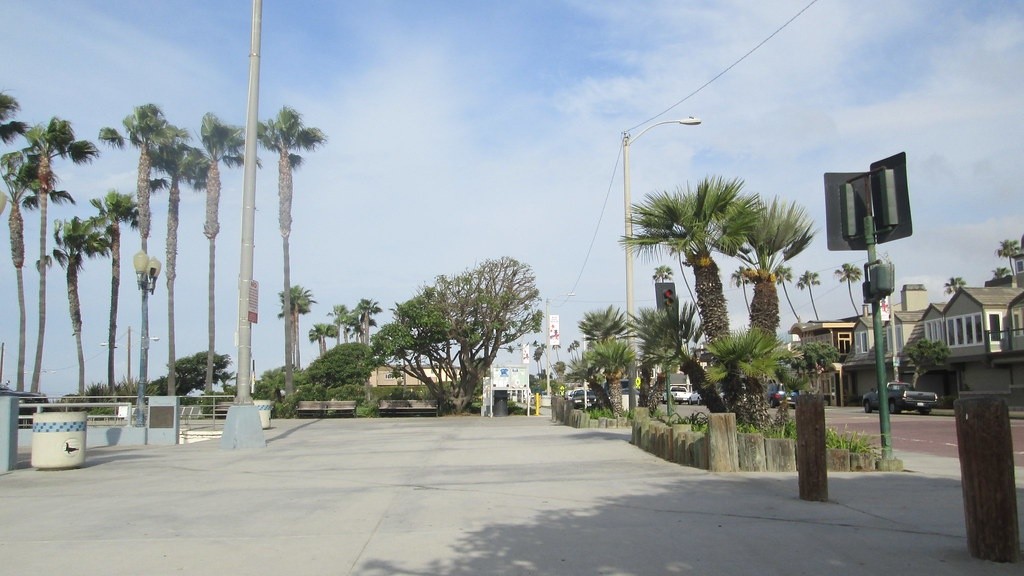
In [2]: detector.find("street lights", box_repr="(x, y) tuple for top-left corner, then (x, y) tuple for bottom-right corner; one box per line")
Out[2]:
(126, 327), (161, 395)
(620, 117), (705, 415)
(132, 249), (163, 427)
(544, 292), (575, 396)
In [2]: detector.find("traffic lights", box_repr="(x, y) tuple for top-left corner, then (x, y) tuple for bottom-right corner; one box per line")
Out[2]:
(654, 281), (676, 309)
(871, 261), (897, 296)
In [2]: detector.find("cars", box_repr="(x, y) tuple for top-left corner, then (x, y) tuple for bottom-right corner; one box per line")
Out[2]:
(768, 381), (799, 407)
(657, 384), (706, 405)
(567, 389), (599, 410)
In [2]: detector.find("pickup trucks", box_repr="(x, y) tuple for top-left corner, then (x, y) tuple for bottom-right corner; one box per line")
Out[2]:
(861, 381), (938, 416)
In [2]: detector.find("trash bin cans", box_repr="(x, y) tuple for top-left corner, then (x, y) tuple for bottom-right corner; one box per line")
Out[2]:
(0, 391), (22, 473)
(254, 399), (272, 428)
(30, 411), (88, 470)
